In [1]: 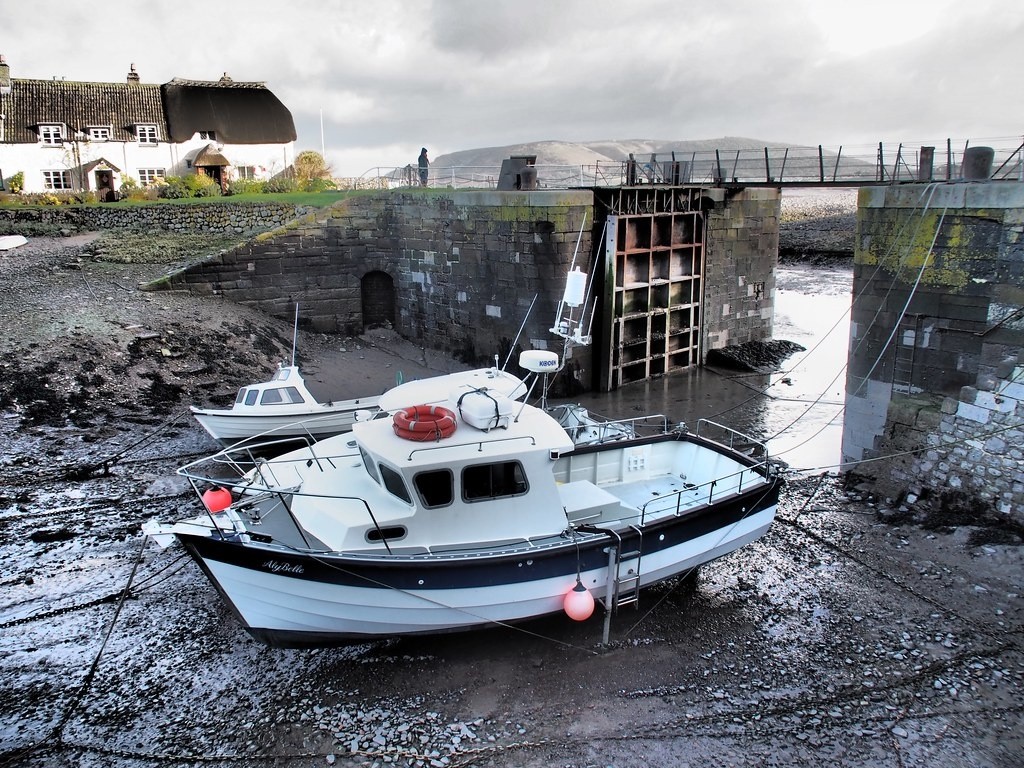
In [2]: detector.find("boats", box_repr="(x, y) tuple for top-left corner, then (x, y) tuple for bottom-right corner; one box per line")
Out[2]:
(140, 210), (788, 650)
(189, 302), (389, 458)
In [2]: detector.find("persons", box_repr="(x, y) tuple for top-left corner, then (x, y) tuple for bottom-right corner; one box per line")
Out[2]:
(626, 153), (636, 187)
(418, 148), (431, 188)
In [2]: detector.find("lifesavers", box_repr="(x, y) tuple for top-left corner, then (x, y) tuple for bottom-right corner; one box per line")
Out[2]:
(392, 404), (457, 443)
